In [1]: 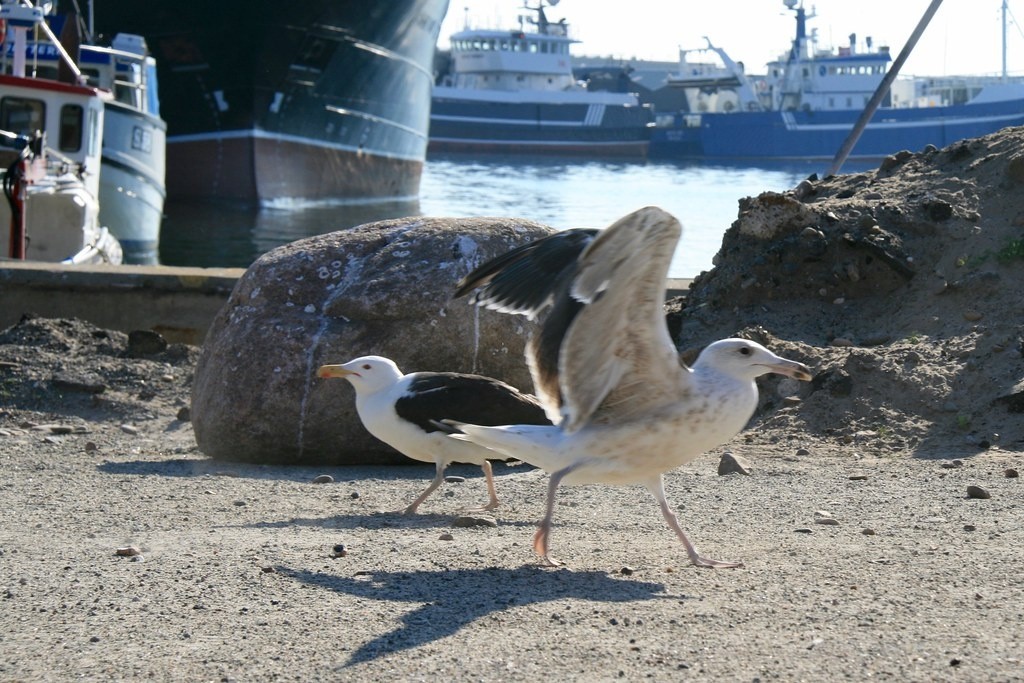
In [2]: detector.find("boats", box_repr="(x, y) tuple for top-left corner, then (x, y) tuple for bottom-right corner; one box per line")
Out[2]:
(0, 71), (123, 266)
(646, 1), (1024, 177)
(430, 1), (657, 157)
(50, 0), (449, 268)
(0, 0), (173, 265)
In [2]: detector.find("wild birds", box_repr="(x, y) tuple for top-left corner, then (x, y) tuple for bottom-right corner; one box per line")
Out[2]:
(316, 356), (553, 514)
(429, 206), (813, 571)
